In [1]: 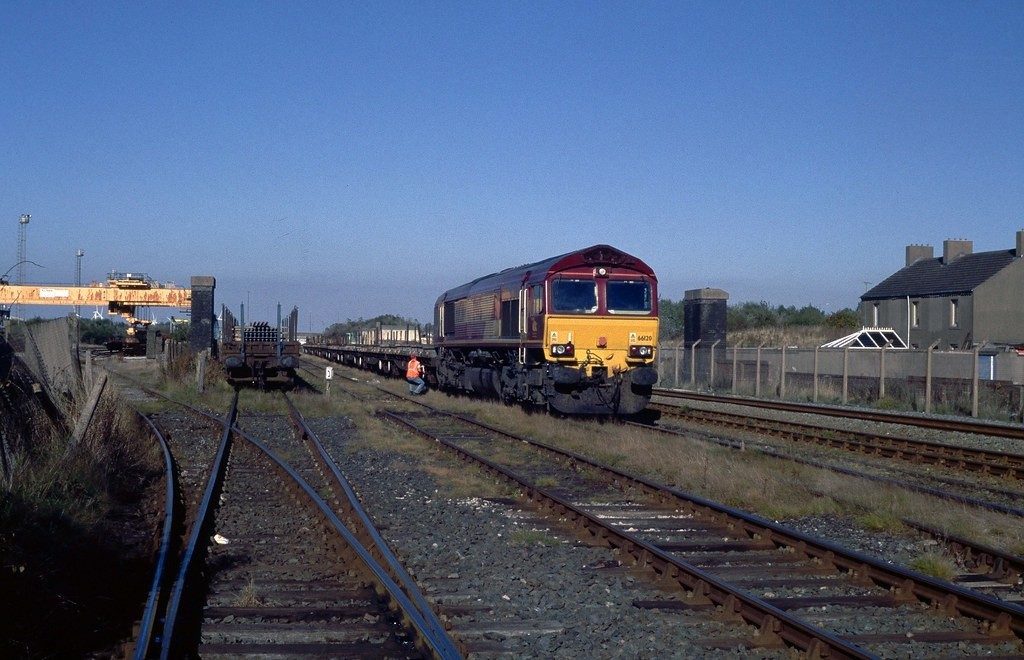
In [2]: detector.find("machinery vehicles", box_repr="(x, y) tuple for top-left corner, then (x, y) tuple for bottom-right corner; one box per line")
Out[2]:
(0, 272), (191, 356)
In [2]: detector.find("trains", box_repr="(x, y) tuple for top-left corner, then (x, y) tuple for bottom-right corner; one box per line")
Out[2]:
(303, 244), (659, 415)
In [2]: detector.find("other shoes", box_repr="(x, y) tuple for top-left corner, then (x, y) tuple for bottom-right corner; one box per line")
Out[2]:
(410, 391), (413, 396)
(413, 392), (420, 396)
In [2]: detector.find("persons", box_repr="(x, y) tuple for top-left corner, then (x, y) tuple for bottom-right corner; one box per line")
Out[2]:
(406, 354), (425, 396)
